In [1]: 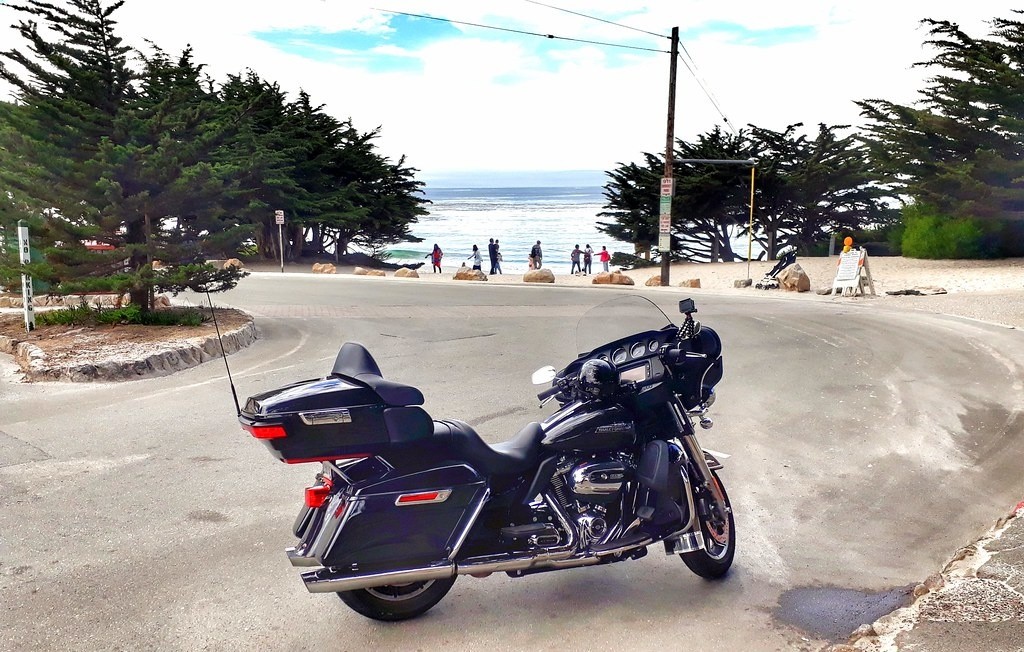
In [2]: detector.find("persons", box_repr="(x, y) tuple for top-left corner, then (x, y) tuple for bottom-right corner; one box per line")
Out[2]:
(488, 238), (502, 275)
(528, 241), (543, 270)
(462, 245), (483, 271)
(429, 244), (443, 273)
(571, 244), (608, 274)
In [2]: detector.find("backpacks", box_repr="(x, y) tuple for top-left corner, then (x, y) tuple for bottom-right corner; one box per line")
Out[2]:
(584, 249), (590, 263)
(604, 252), (610, 261)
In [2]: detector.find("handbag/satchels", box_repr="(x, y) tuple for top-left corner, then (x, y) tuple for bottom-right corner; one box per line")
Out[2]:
(531, 247), (536, 258)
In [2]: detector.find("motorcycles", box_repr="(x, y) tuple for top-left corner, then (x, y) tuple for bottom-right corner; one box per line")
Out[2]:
(238, 293), (736, 623)
